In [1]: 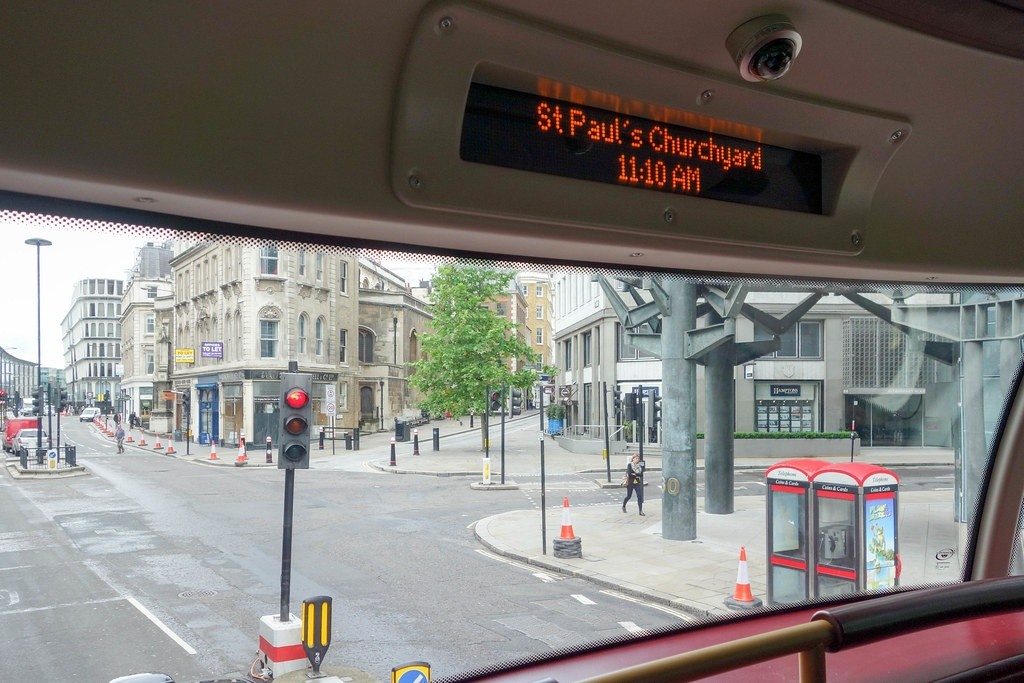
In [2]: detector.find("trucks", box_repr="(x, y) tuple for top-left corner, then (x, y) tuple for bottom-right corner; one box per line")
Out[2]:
(2, 416), (38, 452)
(20, 397), (35, 416)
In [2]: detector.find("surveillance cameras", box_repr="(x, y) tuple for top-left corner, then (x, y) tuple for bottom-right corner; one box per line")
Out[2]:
(723, 16), (802, 83)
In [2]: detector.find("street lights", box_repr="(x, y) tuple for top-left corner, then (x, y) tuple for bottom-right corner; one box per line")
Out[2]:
(23, 238), (53, 467)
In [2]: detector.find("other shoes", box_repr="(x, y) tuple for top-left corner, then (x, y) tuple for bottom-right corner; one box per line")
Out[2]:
(117, 452), (121, 454)
(639, 511), (645, 517)
(622, 506), (626, 512)
(122, 449), (124, 452)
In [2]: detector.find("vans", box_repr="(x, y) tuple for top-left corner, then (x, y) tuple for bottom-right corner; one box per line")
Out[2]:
(79, 407), (101, 422)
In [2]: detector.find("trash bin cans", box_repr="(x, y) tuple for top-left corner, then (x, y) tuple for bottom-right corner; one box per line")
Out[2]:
(395, 420), (410, 442)
(65, 443), (76, 466)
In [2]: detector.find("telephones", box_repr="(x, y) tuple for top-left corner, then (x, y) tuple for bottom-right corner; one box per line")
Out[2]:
(824, 534), (846, 559)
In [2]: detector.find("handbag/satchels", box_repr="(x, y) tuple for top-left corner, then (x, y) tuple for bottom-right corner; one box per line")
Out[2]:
(621, 477), (628, 488)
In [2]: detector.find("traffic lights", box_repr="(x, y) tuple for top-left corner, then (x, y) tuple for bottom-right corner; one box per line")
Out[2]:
(609, 386), (622, 418)
(278, 372), (313, 471)
(182, 389), (190, 411)
(31, 386), (44, 417)
(507, 386), (521, 418)
(56, 383), (68, 410)
(490, 390), (500, 411)
(648, 389), (662, 428)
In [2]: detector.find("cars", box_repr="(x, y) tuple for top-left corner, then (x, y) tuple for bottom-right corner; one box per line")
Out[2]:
(12, 428), (50, 457)
(45, 404), (55, 417)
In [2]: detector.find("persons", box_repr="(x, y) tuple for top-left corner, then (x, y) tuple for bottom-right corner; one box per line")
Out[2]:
(621, 454), (646, 516)
(129, 412), (135, 429)
(113, 424), (125, 454)
(114, 413), (121, 425)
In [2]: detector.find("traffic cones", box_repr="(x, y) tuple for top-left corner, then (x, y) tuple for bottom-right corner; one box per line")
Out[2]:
(208, 440), (220, 460)
(154, 433), (164, 449)
(138, 430), (148, 446)
(553, 495), (583, 559)
(725, 547), (762, 611)
(235, 439), (248, 464)
(125, 427), (135, 442)
(93, 414), (115, 437)
(165, 434), (177, 454)
